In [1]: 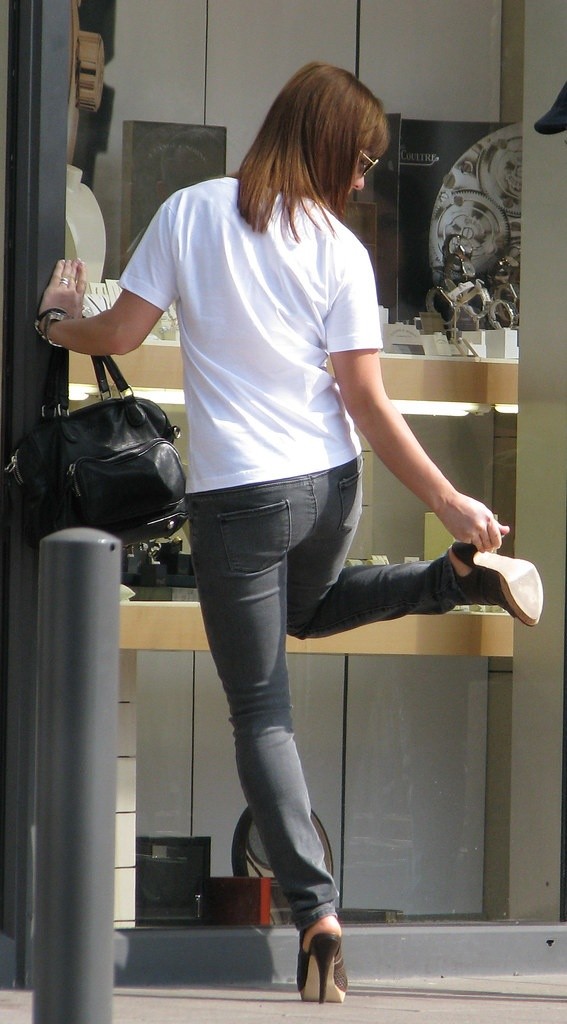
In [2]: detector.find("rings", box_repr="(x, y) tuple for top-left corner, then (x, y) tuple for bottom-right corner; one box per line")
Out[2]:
(60, 278), (69, 285)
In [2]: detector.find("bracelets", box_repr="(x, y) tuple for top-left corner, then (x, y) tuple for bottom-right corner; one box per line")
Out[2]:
(35, 308), (69, 342)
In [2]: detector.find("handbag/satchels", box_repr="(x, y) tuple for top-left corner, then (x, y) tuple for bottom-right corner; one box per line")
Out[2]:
(6, 315), (187, 549)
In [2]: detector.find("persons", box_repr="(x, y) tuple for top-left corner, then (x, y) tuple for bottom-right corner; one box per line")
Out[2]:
(34, 62), (545, 1003)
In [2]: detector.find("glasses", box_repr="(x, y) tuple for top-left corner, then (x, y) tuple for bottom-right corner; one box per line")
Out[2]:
(360, 149), (379, 176)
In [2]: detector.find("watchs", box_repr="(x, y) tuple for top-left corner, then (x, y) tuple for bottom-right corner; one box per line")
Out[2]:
(45, 311), (70, 348)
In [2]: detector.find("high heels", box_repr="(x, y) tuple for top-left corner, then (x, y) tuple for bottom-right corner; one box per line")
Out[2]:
(297, 917), (347, 1004)
(450, 529), (544, 628)
